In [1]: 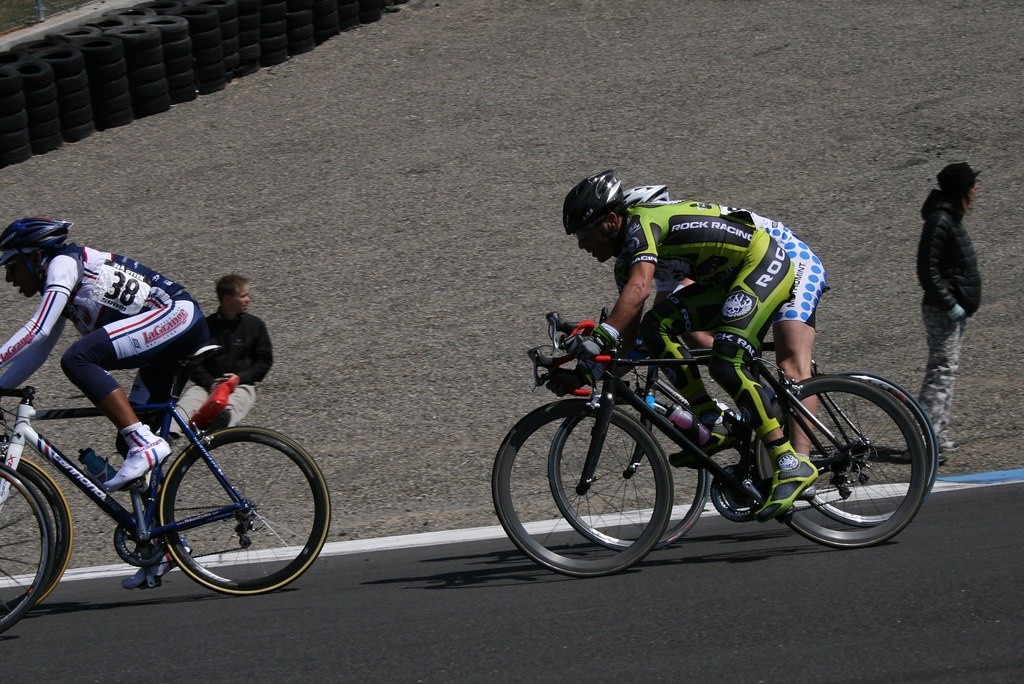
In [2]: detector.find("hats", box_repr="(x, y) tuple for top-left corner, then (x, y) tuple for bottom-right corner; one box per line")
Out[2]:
(936, 162), (982, 196)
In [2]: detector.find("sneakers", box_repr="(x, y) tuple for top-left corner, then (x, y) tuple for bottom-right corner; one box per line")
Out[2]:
(754, 456), (818, 524)
(939, 440), (961, 454)
(669, 410), (741, 467)
(103, 437), (172, 493)
(902, 450), (947, 466)
(121, 535), (193, 589)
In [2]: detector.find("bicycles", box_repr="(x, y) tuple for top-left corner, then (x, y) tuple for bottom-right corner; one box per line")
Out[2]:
(490, 305), (940, 581)
(1, 342), (334, 638)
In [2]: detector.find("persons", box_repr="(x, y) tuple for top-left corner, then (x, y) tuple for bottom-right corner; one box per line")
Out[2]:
(563, 169), (826, 524)
(902, 162), (981, 464)
(158, 275), (273, 437)
(0, 217), (209, 589)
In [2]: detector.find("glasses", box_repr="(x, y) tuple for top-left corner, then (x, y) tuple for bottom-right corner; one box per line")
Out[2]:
(4, 249), (44, 273)
(574, 214), (609, 241)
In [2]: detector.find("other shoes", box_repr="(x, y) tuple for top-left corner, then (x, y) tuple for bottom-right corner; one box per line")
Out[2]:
(796, 485), (816, 501)
(165, 434), (173, 446)
(205, 410), (231, 437)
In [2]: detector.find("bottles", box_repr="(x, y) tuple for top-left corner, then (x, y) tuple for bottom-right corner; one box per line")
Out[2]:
(665, 401), (710, 448)
(78, 447), (126, 492)
(713, 397), (743, 421)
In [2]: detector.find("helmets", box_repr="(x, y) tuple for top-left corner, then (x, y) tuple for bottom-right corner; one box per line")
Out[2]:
(0, 217), (74, 266)
(623, 184), (670, 208)
(562, 170), (624, 235)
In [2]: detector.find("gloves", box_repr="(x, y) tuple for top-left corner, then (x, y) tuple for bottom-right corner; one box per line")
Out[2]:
(945, 303), (967, 323)
(546, 364), (594, 397)
(573, 338), (608, 359)
